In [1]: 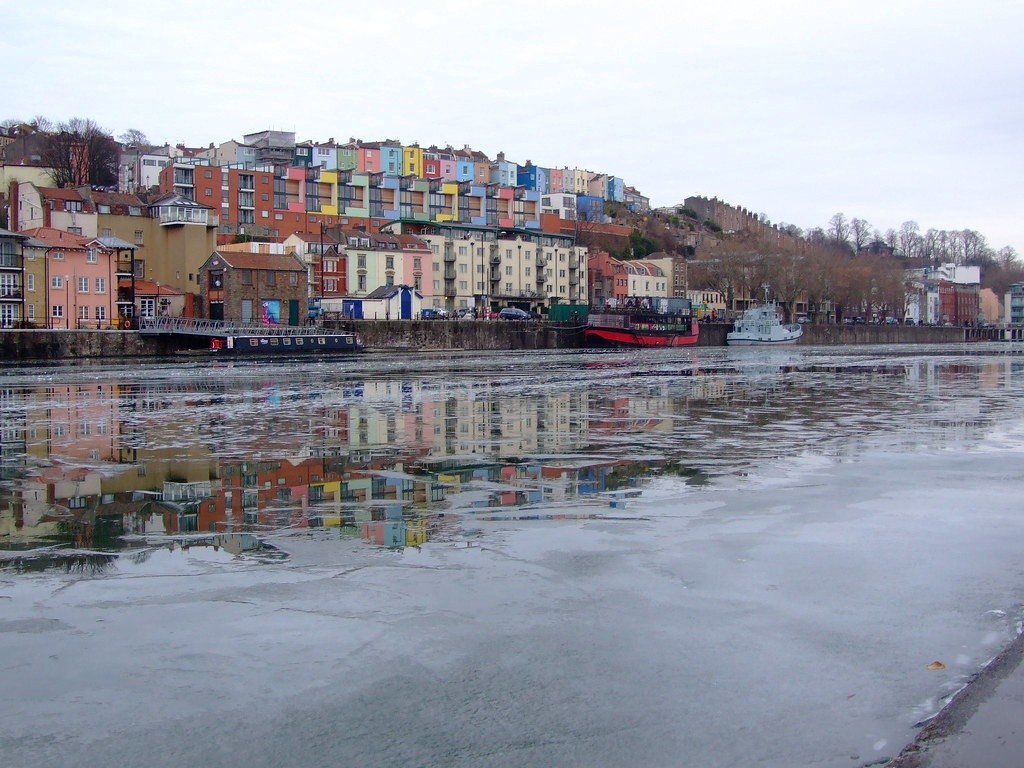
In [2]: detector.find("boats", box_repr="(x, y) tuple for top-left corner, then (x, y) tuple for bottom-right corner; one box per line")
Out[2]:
(727, 281), (804, 345)
(585, 307), (700, 347)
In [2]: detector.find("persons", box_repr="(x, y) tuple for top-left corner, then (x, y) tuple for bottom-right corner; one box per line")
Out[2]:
(919, 319), (923, 326)
(474, 305), (478, 320)
(965, 319), (969, 327)
(852, 316), (857, 323)
(705, 307), (718, 323)
(971, 319), (973, 327)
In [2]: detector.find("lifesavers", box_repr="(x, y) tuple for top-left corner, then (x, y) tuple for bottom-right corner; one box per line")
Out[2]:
(125, 321), (130, 327)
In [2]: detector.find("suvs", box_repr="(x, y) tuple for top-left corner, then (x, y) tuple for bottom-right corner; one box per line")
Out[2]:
(453, 309), (466, 318)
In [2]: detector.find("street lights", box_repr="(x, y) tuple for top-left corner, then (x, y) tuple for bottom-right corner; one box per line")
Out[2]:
(64, 275), (70, 330)
(156, 281), (161, 328)
(215, 281), (222, 329)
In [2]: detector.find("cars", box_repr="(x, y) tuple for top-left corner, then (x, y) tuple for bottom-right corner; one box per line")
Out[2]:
(500, 308), (531, 320)
(435, 308), (451, 318)
(484, 311), (498, 320)
(421, 309), (449, 321)
